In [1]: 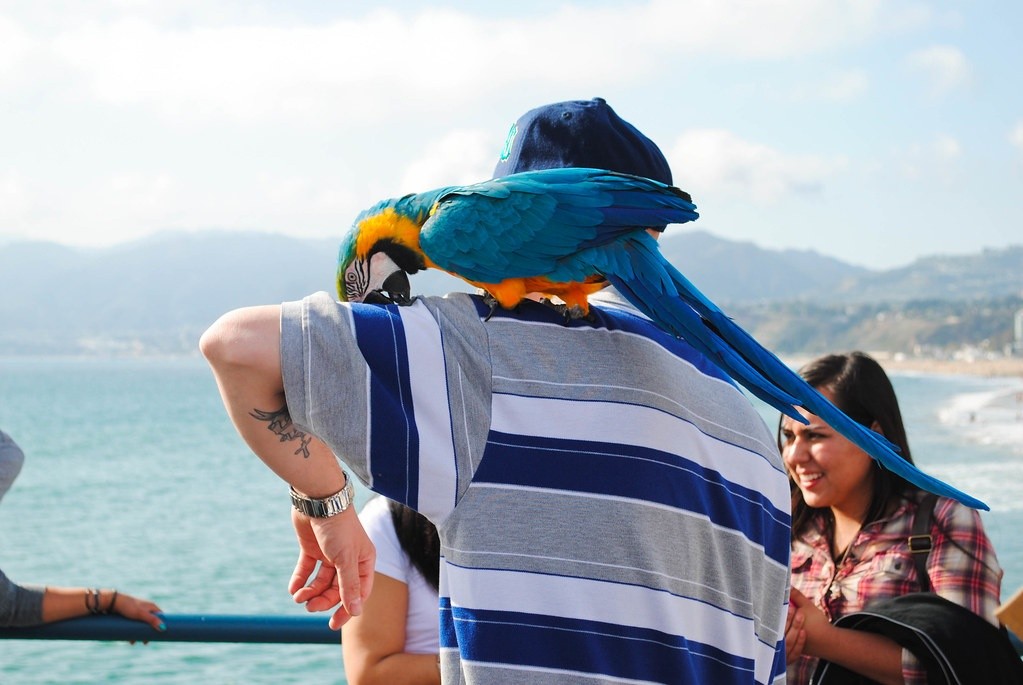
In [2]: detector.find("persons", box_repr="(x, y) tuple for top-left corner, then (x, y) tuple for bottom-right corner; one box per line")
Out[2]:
(775, 349), (1005, 685)
(0, 430), (167, 646)
(200, 95), (791, 685)
(338, 494), (441, 685)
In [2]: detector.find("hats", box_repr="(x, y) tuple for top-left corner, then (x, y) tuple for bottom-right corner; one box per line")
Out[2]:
(491, 98), (673, 232)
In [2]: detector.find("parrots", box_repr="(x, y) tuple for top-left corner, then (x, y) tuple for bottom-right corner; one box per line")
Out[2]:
(337, 166), (993, 514)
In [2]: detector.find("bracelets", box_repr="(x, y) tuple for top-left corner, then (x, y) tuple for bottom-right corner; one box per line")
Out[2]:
(287, 473), (353, 519)
(85, 587), (92, 611)
(104, 587), (118, 612)
(92, 588), (100, 613)
